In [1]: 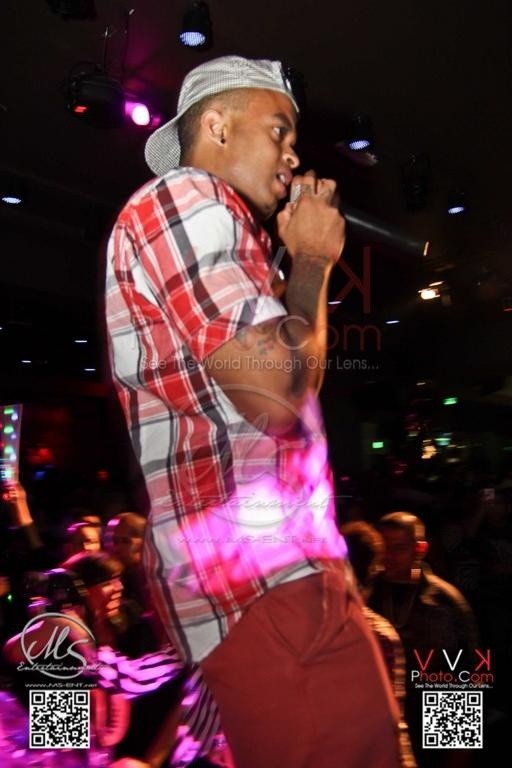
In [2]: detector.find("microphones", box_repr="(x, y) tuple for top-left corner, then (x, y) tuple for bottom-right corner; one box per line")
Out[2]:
(287, 184), (431, 258)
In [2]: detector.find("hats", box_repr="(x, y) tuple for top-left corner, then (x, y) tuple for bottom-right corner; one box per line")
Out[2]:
(143, 55), (300, 177)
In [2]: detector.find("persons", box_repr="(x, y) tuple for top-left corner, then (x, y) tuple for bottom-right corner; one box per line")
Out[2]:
(99, 48), (420, 767)
